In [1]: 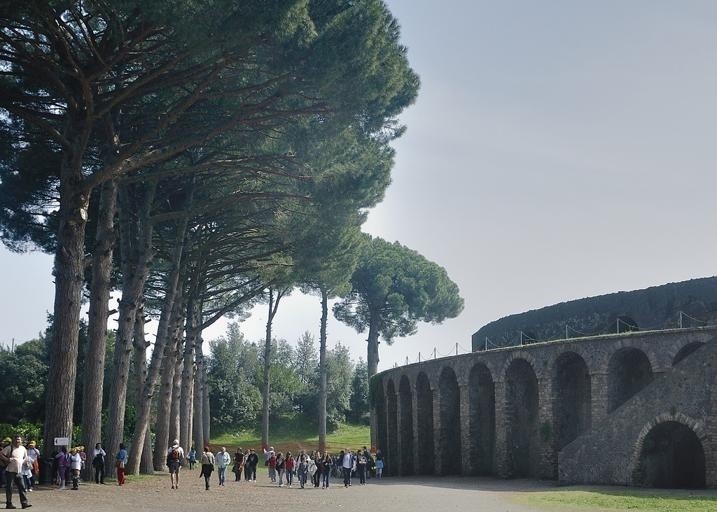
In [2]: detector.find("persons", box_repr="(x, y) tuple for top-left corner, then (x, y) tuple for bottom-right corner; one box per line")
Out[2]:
(215, 446), (231, 486)
(168, 439), (184, 490)
(93, 443), (106, 484)
(115, 442), (129, 486)
(188, 446), (197, 470)
(0, 436), (33, 509)
(48, 445), (86, 492)
(263, 446), (384, 490)
(199, 447), (215, 491)
(2, 438), (40, 491)
(233, 446), (258, 483)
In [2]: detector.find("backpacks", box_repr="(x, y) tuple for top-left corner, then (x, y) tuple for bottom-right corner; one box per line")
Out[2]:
(170, 449), (180, 460)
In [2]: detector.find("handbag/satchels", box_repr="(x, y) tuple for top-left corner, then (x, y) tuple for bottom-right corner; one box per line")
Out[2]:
(209, 462), (214, 471)
(265, 460), (270, 466)
(186, 455), (191, 460)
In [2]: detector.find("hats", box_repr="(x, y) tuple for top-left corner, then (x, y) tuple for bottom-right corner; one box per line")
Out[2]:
(69, 444), (86, 453)
(3, 436), (12, 443)
(29, 440), (37, 446)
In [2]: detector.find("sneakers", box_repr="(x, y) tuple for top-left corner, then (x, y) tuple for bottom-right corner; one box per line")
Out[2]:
(205, 479), (367, 490)
(22, 502), (32, 509)
(171, 485), (179, 489)
(25, 488), (32, 492)
(6, 503), (16, 509)
